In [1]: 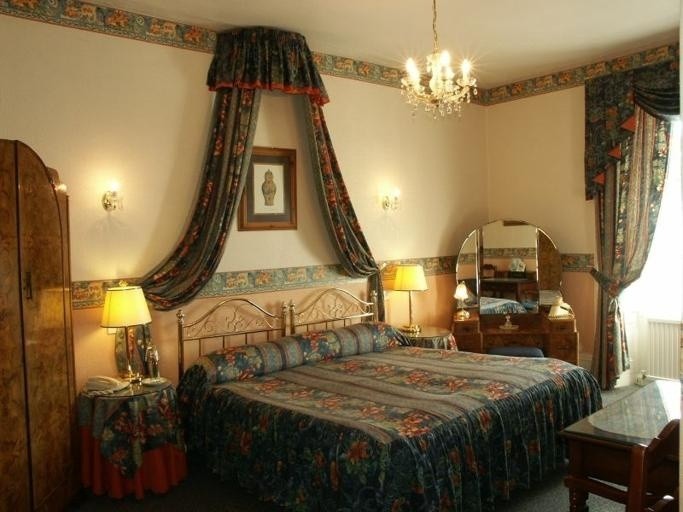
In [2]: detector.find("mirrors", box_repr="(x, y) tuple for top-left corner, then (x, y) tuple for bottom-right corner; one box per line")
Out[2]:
(455, 218), (563, 318)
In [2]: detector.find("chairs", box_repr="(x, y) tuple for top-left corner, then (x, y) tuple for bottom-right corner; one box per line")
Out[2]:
(625, 419), (683, 512)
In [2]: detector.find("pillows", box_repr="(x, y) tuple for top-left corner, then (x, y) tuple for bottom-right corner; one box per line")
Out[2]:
(185, 320), (411, 385)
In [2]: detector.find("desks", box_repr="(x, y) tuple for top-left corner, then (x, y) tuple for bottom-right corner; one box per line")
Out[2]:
(557, 379), (683, 512)
(75, 375), (187, 501)
(451, 298), (580, 366)
(480, 276), (537, 301)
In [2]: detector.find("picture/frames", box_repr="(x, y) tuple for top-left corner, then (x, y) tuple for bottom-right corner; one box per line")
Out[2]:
(237, 146), (298, 232)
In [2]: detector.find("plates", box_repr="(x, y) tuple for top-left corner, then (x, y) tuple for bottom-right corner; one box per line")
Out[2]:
(142, 377), (167, 387)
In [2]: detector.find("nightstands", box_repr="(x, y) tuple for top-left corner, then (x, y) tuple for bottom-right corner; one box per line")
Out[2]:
(398, 326), (459, 351)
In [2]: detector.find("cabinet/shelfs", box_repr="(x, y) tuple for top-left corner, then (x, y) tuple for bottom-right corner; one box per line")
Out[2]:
(0, 139), (77, 512)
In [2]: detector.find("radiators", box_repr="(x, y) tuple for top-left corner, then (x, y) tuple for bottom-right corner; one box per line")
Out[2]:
(634, 318), (683, 386)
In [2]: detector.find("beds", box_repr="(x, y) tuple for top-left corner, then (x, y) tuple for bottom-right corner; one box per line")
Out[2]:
(176, 287), (603, 512)
(479, 296), (528, 316)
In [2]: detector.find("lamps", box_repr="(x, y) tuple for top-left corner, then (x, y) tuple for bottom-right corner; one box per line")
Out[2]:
(102, 181), (125, 213)
(398, 0), (480, 118)
(383, 188), (402, 212)
(100, 285), (153, 384)
(453, 281), (471, 322)
(390, 263), (429, 333)
